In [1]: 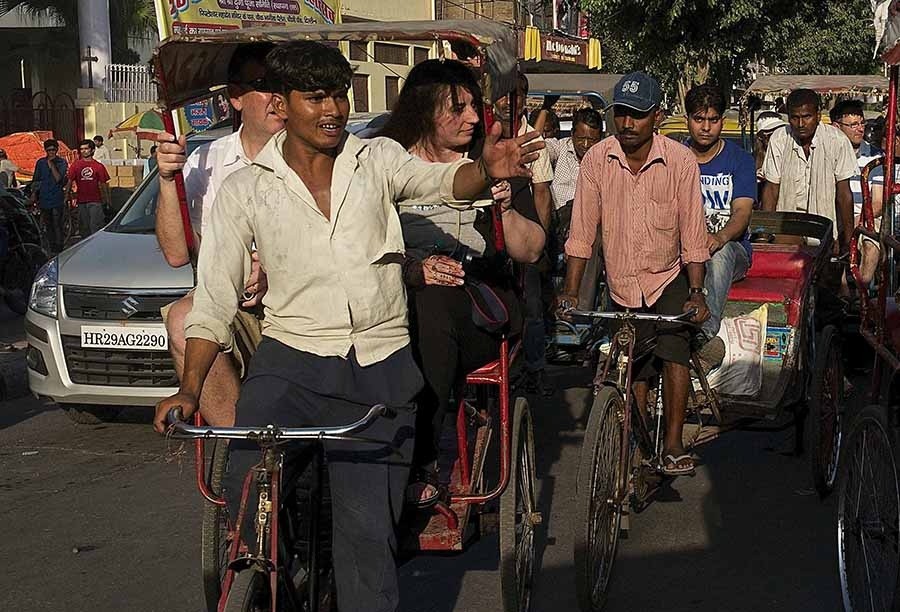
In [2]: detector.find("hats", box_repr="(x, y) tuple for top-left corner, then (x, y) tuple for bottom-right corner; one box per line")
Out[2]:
(602, 71), (663, 113)
(756, 117), (790, 134)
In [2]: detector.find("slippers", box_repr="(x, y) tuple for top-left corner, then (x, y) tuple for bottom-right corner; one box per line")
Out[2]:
(403, 464), (443, 509)
(661, 454), (695, 476)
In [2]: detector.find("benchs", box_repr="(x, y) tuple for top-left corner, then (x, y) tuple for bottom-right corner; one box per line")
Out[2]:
(730, 247), (813, 303)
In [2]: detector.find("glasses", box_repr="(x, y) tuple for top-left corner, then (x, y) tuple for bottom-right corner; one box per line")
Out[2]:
(837, 121), (867, 129)
(760, 131), (772, 138)
(47, 148), (57, 152)
(237, 76), (275, 93)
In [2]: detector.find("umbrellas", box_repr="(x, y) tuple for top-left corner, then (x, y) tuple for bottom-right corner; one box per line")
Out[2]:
(109, 108), (166, 141)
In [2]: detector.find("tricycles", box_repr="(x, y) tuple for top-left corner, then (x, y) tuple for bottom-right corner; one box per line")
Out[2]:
(154, 183), (540, 610)
(557, 207), (834, 608)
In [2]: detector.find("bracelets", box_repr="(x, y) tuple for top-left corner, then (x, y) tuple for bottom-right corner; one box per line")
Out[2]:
(477, 155), (495, 186)
(401, 258), (416, 279)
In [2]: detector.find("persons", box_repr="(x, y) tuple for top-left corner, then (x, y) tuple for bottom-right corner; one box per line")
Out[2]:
(760, 88), (856, 320)
(156, 42), (287, 429)
(93, 135), (110, 160)
(549, 73), (712, 477)
(678, 84), (756, 344)
(882, 129), (900, 261)
(63, 139), (111, 239)
(364, 59), (544, 509)
(544, 106), (604, 321)
(830, 99), (886, 312)
(757, 110), (785, 183)
(216, 94), (231, 121)
(0, 148), (34, 187)
(152, 39), (546, 612)
(489, 71), (555, 233)
(25, 140), (68, 252)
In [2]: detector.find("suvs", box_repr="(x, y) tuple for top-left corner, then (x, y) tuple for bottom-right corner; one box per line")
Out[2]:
(21, 110), (400, 426)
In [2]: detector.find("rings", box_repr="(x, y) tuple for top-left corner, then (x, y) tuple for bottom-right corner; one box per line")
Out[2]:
(432, 263), (437, 272)
(253, 283), (257, 293)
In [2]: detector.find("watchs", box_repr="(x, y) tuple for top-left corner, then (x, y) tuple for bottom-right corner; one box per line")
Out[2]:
(689, 287), (709, 296)
